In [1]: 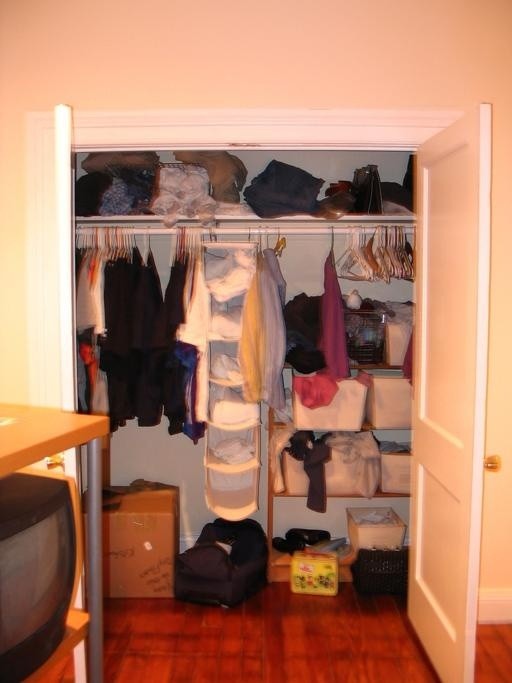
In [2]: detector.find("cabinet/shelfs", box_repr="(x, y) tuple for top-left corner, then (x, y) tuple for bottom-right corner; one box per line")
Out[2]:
(265, 362), (410, 582)
(1, 401), (113, 683)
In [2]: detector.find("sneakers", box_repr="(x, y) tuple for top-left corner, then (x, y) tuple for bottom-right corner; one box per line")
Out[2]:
(272, 529), (330, 554)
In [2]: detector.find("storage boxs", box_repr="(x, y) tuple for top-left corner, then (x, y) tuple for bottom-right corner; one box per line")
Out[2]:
(291, 375), (369, 433)
(279, 445), (378, 497)
(372, 439), (409, 501)
(83, 478), (181, 602)
(347, 503), (407, 551)
(361, 371), (416, 430)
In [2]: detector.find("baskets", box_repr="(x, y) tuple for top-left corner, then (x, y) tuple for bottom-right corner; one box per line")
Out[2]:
(353, 548), (408, 580)
(104, 162), (211, 179)
(343, 305), (386, 364)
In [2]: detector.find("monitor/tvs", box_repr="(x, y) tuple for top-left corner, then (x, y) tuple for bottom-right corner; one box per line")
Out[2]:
(1, 472), (77, 683)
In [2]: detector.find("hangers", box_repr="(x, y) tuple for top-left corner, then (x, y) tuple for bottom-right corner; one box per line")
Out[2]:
(321, 222), (419, 286)
(75, 227), (291, 269)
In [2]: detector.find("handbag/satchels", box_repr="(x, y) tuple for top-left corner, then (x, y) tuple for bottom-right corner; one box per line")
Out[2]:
(350, 162), (386, 216)
(177, 514), (270, 610)
